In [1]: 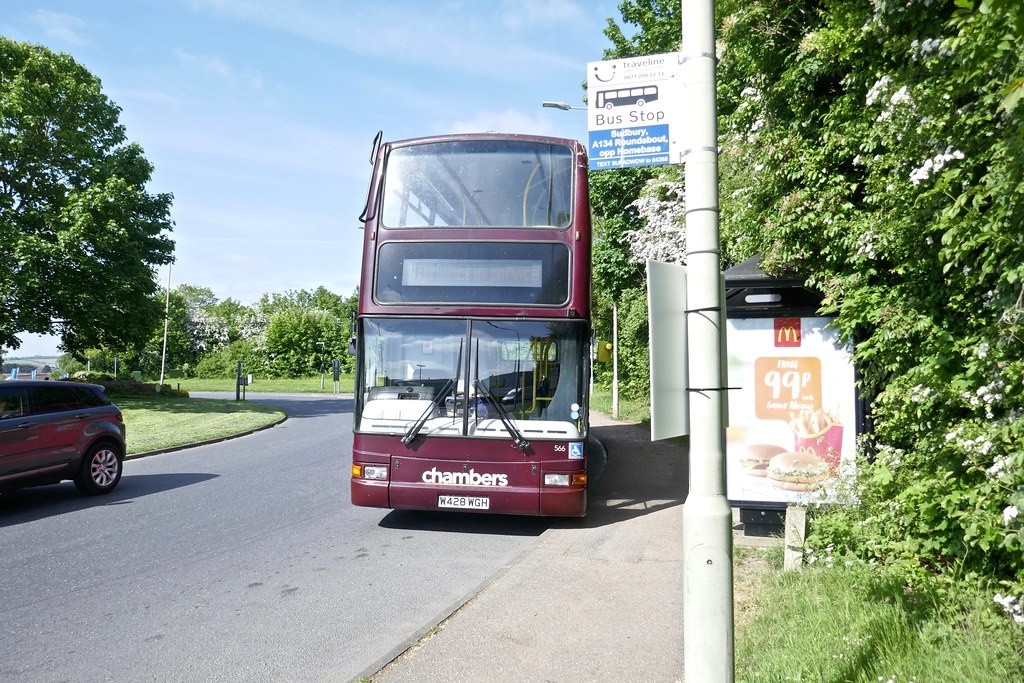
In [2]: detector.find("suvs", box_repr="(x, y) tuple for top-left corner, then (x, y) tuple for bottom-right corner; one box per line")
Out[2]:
(0, 378), (126, 494)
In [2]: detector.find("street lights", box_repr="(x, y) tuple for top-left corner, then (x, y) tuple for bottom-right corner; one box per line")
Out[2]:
(542, 101), (621, 420)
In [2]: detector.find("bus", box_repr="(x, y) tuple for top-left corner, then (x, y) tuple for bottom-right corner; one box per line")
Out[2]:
(350, 130), (613, 519)
